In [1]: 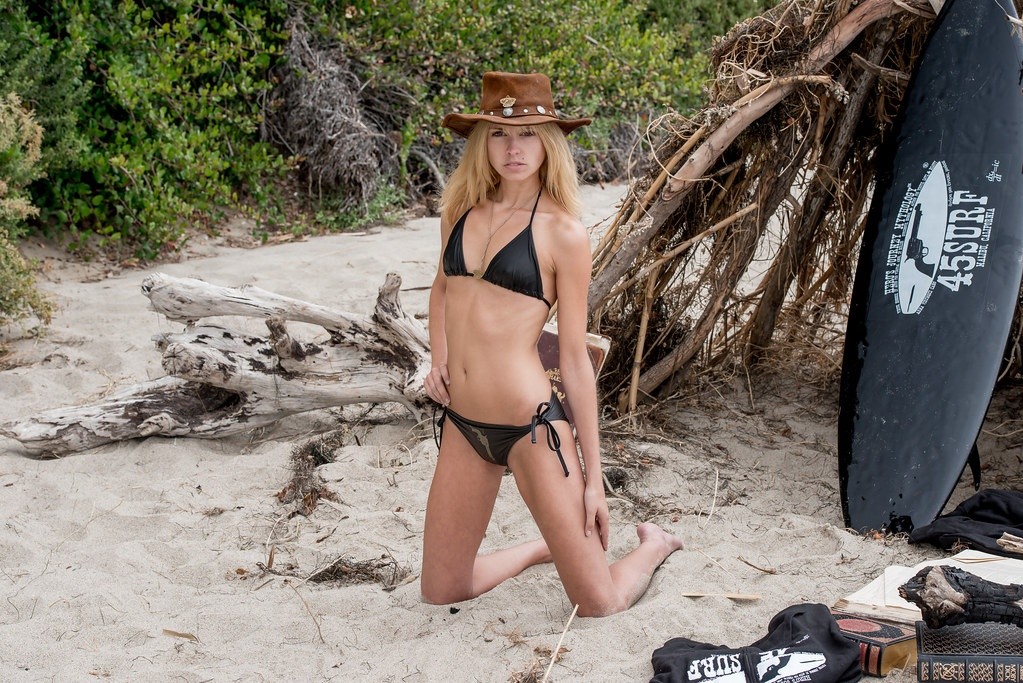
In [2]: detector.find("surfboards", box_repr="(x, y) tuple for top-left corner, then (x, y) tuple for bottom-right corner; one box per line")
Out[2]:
(837, 0), (1023, 540)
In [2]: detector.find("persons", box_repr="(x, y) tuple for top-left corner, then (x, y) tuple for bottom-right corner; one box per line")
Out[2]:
(417, 69), (683, 617)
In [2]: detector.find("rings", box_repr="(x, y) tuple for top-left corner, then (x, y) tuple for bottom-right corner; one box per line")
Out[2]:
(439, 364), (447, 368)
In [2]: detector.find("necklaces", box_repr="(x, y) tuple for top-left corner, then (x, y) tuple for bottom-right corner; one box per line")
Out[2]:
(473, 180), (542, 280)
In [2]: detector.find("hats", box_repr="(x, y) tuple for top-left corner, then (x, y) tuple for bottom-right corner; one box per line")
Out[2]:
(440, 71), (592, 139)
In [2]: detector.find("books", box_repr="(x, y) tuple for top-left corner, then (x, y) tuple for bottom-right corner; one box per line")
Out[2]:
(829, 610), (917, 678)
(913, 619), (1023, 683)
(833, 549), (1023, 627)
